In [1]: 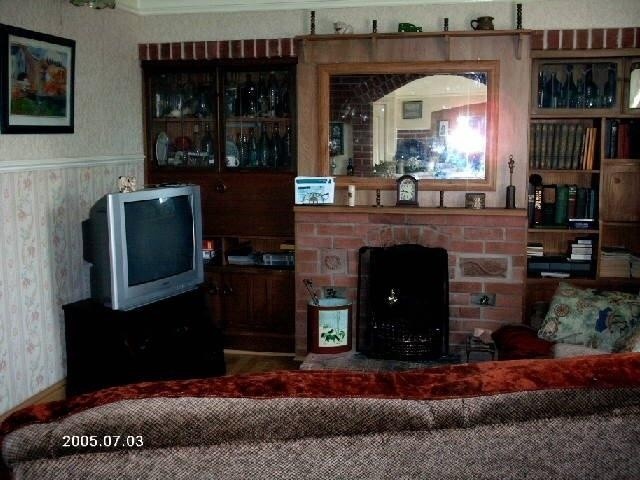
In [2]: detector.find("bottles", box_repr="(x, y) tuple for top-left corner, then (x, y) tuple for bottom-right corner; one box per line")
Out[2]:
(539, 62), (617, 109)
(151, 70), (293, 118)
(397, 157), (404, 175)
(347, 159), (353, 175)
(192, 117), (295, 169)
(348, 184), (356, 206)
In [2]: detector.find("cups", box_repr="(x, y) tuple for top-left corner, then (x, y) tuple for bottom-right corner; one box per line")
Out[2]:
(174, 137), (191, 152)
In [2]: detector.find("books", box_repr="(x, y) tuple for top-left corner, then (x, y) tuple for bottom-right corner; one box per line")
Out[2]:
(526, 119), (640, 278)
(263, 240), (295, 264)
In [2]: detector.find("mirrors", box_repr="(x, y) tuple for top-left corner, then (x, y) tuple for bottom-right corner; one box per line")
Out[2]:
(317, 59), (501, 192)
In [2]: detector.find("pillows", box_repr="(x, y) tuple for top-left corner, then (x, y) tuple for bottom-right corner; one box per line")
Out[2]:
(537, 282), (640, 353)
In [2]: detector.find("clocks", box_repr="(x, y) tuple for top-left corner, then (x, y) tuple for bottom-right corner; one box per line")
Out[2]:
(395, 174), (420, 208)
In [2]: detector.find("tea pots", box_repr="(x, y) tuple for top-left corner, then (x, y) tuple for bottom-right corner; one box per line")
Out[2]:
(471, 16), (495, 30)
(333, 22), (353, 34)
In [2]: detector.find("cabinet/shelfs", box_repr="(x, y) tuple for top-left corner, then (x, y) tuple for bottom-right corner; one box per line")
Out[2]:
(531, 48), (640, 116)
(61, 292), (227, 400)
(528, 118), (640, 281)
(202, 264), (294, 355)
(144, 174), (295, 239)
(141, 56), (297, 174)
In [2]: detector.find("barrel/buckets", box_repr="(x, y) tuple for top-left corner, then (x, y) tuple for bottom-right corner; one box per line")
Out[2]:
(307, 298), (353, 354)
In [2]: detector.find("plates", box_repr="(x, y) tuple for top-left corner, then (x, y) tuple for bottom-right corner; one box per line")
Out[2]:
(155, 131), (170, 164)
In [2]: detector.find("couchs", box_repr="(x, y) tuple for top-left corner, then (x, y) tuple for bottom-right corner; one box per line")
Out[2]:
(491, 280), (640, 361)
(0, 352), (640, 479)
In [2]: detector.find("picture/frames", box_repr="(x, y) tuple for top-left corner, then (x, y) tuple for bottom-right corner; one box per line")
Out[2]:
(402, 100), (423, 119)
(1, 23), (80, 136)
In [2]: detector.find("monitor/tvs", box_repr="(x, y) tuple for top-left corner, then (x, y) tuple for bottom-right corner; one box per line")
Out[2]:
(82, 184), (204, 312)
